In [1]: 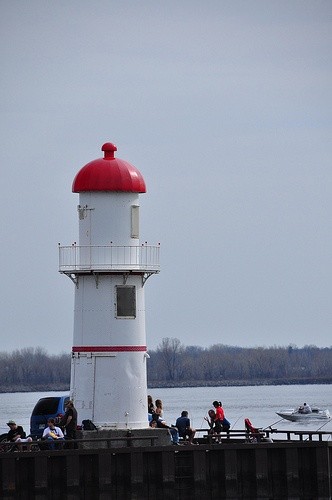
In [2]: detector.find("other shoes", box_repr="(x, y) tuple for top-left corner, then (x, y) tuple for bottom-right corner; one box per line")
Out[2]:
(189, 442), (195, 445)
(173, 442), (181, 445)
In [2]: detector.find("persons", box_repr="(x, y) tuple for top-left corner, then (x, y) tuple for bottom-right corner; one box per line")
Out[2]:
(147, 395), (155, 414)
(1, 419), (33, 452)
(211, 401), (225, 444)
(153, 408), (181, 445)
(54, 414), (63, 427)
(175, 411), (196, 446)
(60, 400), (79, 450)
(203, 409), (231, 444)
(42, 418), (67, 451)
(150, 399), (163, 428)
(300, 403), (312, 414)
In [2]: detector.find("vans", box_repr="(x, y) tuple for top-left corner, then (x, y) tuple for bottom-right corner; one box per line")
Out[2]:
(30, 395), (70, 440)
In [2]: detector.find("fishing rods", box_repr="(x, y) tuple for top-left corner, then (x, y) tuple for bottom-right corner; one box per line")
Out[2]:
(229, 414), (244, 434)
(304, 418), (332, 442)
(263, 400), (320, 430)
(196, 411), (208, 441)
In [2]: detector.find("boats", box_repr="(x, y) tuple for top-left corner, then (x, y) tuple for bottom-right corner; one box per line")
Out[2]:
(275, 407), (330, 422)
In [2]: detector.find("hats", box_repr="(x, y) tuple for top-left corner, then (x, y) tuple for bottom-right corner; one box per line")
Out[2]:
(6, 421), (16, 425)
(152, 413), (160, 417)
